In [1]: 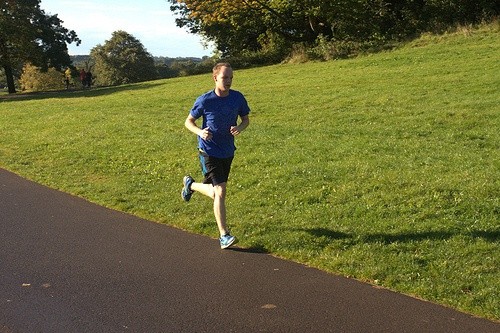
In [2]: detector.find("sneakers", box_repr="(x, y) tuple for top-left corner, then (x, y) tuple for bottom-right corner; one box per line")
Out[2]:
(181, 174), (195, 202)
(218, 231), (239, 250)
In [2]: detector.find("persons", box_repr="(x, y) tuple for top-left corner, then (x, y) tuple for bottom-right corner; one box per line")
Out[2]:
(180, 62), (252, 250)
